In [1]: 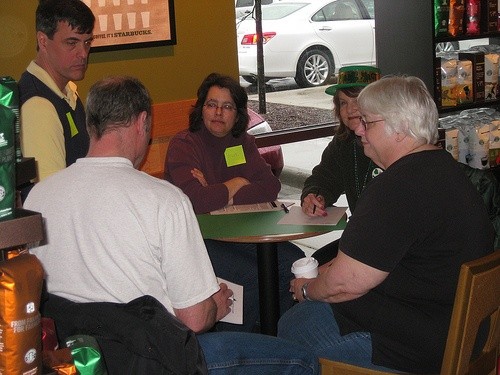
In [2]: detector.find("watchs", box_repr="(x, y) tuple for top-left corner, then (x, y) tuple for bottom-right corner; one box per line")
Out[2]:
(301, 278), (316, 302)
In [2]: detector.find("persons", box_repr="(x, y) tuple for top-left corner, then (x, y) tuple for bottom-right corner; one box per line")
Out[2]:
(19, 65), (494, 375)
(18, 0), (95, 206)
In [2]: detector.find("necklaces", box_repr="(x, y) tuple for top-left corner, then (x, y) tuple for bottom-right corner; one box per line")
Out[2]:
(354, 132), (371, 199)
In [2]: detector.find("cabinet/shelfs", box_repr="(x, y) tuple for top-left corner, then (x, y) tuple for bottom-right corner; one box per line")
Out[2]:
(435, 31), (500, 112)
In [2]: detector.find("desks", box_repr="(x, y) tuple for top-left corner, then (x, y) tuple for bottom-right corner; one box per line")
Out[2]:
(197, 200), (347, 337)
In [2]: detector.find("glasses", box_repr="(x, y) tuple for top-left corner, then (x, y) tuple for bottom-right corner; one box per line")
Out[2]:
(203, 103), (237, 113)
(358, 116), (386, 130)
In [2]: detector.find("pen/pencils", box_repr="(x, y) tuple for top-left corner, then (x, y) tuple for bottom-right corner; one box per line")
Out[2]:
(228, 297), (236, 301)
(313, 190), (319, 214)
(281, 203), (289, 214)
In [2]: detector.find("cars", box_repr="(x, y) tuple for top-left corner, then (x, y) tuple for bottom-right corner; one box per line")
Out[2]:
(235, 0), (281, 20)
(235, 0), (489, 88)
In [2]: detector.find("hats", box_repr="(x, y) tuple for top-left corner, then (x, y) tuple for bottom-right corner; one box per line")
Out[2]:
(324, 65), (381, 95)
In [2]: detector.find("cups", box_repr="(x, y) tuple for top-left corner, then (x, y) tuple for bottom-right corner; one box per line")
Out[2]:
(290, 257), (318, 279)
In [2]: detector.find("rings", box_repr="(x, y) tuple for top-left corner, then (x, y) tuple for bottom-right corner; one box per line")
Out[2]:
(293, 295), (296, 299)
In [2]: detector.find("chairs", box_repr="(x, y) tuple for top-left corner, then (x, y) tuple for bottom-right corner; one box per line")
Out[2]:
(319, 249), (500, 375)
